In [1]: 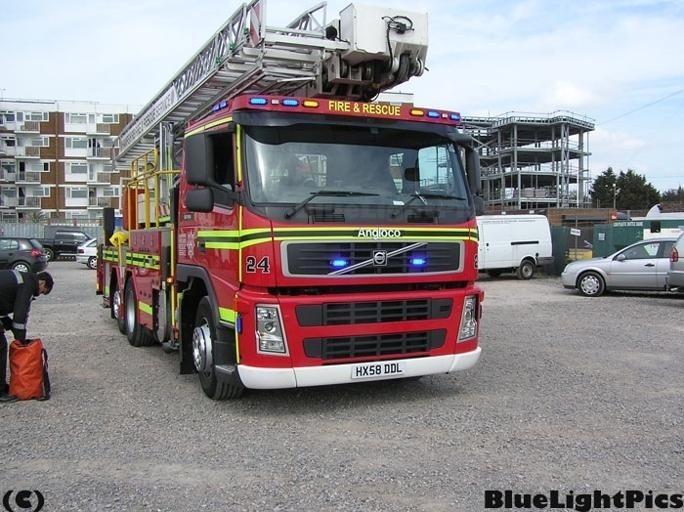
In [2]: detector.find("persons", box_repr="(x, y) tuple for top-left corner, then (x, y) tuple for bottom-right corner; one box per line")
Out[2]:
(0, 269), (54, 401)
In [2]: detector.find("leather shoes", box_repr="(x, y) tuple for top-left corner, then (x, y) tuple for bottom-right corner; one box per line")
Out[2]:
(0, 394), (18, 402)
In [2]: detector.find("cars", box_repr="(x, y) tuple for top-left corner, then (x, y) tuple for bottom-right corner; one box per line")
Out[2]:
(75, 237), (97, 270)
(0, 236), (48, 273)
(560, 237), (678, 298)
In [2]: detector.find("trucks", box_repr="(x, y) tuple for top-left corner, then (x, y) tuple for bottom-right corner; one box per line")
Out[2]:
(475, 214), (555, 279)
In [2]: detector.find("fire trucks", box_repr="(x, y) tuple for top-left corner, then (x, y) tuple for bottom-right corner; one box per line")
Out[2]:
(96, 1), (483, 402)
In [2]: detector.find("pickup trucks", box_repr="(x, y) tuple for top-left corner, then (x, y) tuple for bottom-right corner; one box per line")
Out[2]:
(26, 230), (97, 263)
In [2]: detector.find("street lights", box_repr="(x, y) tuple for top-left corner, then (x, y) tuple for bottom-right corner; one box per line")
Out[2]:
(605, 183), (620, 209)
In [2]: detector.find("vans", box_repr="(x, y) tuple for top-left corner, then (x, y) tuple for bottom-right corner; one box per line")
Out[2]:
(664, 231), (684, 293)
